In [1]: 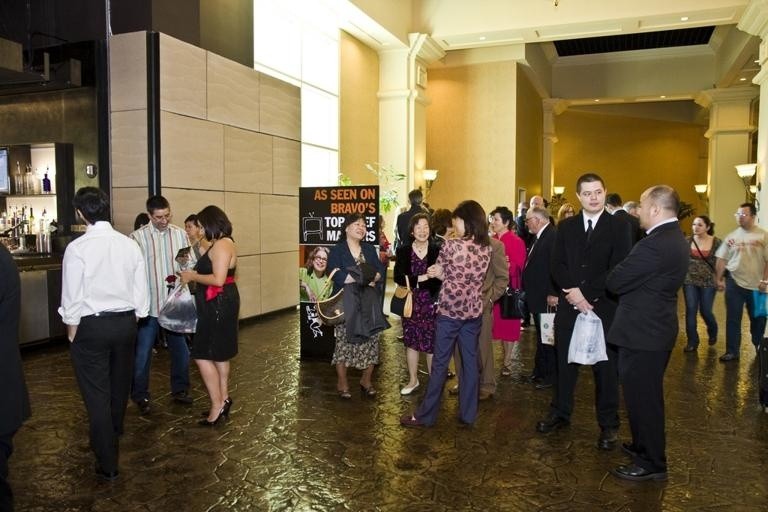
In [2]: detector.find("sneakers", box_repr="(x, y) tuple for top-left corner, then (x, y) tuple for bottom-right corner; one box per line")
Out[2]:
(400, 414), (428, 429)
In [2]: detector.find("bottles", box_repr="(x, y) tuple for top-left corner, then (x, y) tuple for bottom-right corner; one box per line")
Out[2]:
(0, 206), (53, 254)
(14, 161), (50, 194)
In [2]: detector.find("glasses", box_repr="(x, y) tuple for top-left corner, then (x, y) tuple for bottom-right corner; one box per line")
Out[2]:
(150, 213), (173, 221)
(314, 256), (327, 263)
(734, 213), (750, 217)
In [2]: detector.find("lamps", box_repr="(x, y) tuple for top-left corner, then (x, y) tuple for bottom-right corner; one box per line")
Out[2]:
(694, 185), (709, 203)
(554, 186), (567, 205)
(419, 169), (440, 191)
(749, 182), (761, 193)
(734, 163), (761, 211)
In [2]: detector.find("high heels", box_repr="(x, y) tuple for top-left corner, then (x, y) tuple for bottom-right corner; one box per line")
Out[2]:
(198, 397), (233, 426)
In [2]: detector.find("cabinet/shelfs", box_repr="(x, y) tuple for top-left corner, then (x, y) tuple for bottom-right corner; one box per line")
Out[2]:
(0, 144), (74, 255)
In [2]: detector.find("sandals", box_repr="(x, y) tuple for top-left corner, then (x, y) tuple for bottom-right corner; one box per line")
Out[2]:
(337, 384), (351, 401)
(359, 379), (377, 398)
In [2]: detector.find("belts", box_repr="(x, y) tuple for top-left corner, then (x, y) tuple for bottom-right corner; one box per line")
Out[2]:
(92, 310), (135, 318)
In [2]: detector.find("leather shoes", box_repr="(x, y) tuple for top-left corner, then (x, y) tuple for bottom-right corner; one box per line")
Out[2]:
(684, 336), (717, 353)
(621, 440), (635, 456)
(719, 353), (741, 361)
(174, 390), (194, 405)
(501, 362), (514, 375)
(449, 383), (491, 400)
(95, 460), (119, 480)
(138, 395), (153, 416)
(522, 372), (554, 389)
(612, 461), (667, 482)
(400, 380), (421, 396)
(598, 428), (618, 451)
(536, 414), (569, 433)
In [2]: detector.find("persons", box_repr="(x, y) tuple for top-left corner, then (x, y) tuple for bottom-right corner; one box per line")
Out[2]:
(378, 192), (768, 415)
(298, 246), (334, 303)
(184, 213), (213, 263)
(134, 213), (149, 233)
(178, 204), (240, 426)
(326, 214), (386, 400)
(605, 186), (690, 481)
(401, 200), (491, 427)
(537, 173), (631, 450)
(58, 186), (152, 481)
(0, 242), (31, 512)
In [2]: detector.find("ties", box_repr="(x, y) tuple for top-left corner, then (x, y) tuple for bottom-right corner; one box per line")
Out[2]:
(586, 219), (593, 237)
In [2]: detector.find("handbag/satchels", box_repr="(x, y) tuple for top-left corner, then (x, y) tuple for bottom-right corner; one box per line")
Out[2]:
(391, 286), (413, 318)
(540, 311), (556, 346)
(500, 286), (529, 320)
(316, 287), (347, 327)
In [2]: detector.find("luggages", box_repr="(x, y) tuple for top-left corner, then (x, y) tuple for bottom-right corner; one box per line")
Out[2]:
(757, 337), (768, 412)
